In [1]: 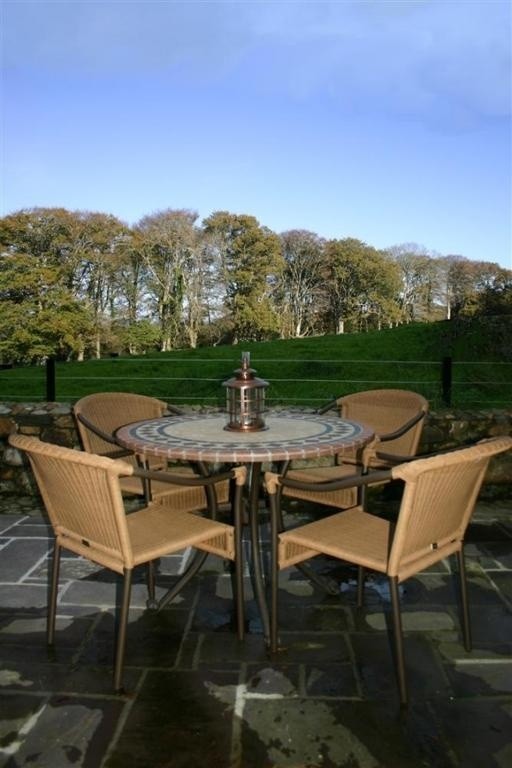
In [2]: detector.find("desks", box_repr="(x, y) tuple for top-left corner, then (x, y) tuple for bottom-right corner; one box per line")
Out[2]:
(113, 408), (376, 648)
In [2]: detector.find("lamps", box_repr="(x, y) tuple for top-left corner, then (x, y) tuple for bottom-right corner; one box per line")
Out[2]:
(220, 350), (270, 433)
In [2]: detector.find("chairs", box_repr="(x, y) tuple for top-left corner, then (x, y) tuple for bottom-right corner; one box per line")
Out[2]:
(8, 433), (237, 695)
(71, 391), (234, 574)
(274, 388), (431, 509)
(271, 434), (512, 706)
